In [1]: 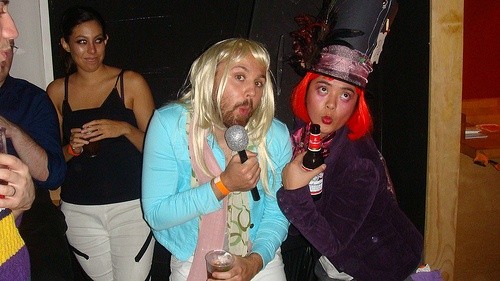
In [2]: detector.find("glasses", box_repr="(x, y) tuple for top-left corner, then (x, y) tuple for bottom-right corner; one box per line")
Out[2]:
(10, 44), (18, 54)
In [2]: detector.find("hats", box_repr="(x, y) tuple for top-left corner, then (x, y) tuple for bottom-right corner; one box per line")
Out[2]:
(289, 0), (400, 87)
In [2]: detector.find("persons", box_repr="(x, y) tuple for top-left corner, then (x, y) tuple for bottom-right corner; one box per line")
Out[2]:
(0, 0), (36, 281)
(46, 6), (156, 281)
(0, 38), (66, 281)
(275, 45), (424, 281)
(141, 37), (291, 281)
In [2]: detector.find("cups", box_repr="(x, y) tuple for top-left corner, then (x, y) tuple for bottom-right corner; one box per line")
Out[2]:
(204, 250), (234, 280)
(0, 128), (9, 186)
(84, 129), (100, 157)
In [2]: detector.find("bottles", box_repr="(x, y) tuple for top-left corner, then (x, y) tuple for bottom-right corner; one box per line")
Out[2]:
(303, 124), (324, 199)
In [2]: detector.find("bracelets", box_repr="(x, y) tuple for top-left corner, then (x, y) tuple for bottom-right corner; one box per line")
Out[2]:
(214, 176), (231, 195)
(69, 145), (84, 156)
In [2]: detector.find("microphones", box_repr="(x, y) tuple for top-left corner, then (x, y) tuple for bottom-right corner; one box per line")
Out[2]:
(225, 125), (260, 201)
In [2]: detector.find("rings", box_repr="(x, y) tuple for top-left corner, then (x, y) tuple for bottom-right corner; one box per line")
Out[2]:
(8, 185), (16, 197)
